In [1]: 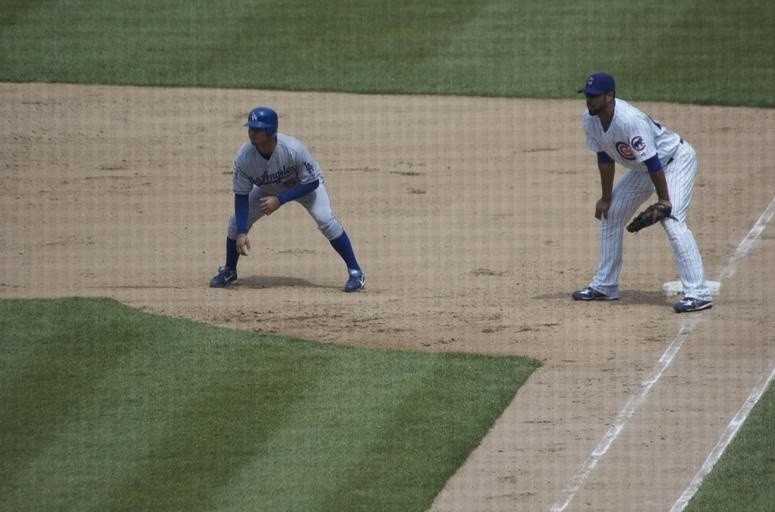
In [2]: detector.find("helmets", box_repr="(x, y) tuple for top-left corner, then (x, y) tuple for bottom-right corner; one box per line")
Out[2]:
(244, 107), (278, 137)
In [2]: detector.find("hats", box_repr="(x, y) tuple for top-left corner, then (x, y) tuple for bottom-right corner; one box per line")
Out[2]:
(578, 73), (615, 95)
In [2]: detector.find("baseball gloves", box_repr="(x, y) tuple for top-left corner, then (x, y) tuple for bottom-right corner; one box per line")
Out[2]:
(626, 204), (678, 232)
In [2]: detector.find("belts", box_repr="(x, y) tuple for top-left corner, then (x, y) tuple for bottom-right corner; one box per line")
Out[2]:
(667, 137), (684, 164)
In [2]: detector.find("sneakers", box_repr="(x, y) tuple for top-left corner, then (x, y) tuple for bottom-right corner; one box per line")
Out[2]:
(572, 286), (620, 301)
(345, 270), (366, 293)
(209, 266), (238, 287)
(673, 298), (713, 312)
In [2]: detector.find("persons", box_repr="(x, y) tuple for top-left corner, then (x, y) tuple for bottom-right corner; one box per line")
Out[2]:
(210, 106), (364, 293)
(571, 72), (715, 311)
